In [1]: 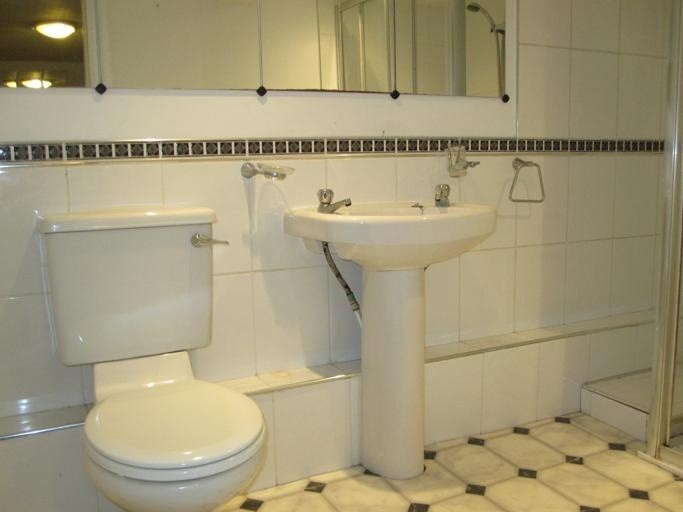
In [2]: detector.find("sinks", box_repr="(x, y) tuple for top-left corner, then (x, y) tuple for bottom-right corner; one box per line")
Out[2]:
(283, 199), (497, 247)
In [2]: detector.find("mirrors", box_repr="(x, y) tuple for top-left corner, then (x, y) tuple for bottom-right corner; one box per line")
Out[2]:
(0, 1), (511, 104)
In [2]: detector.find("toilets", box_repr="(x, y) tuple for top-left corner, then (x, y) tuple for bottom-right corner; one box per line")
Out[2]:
(35, 206), (266, 511)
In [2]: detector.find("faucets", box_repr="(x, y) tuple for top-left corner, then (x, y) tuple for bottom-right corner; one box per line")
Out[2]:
(434, 184), (450, 207)
(316, 188), (351, 212)
